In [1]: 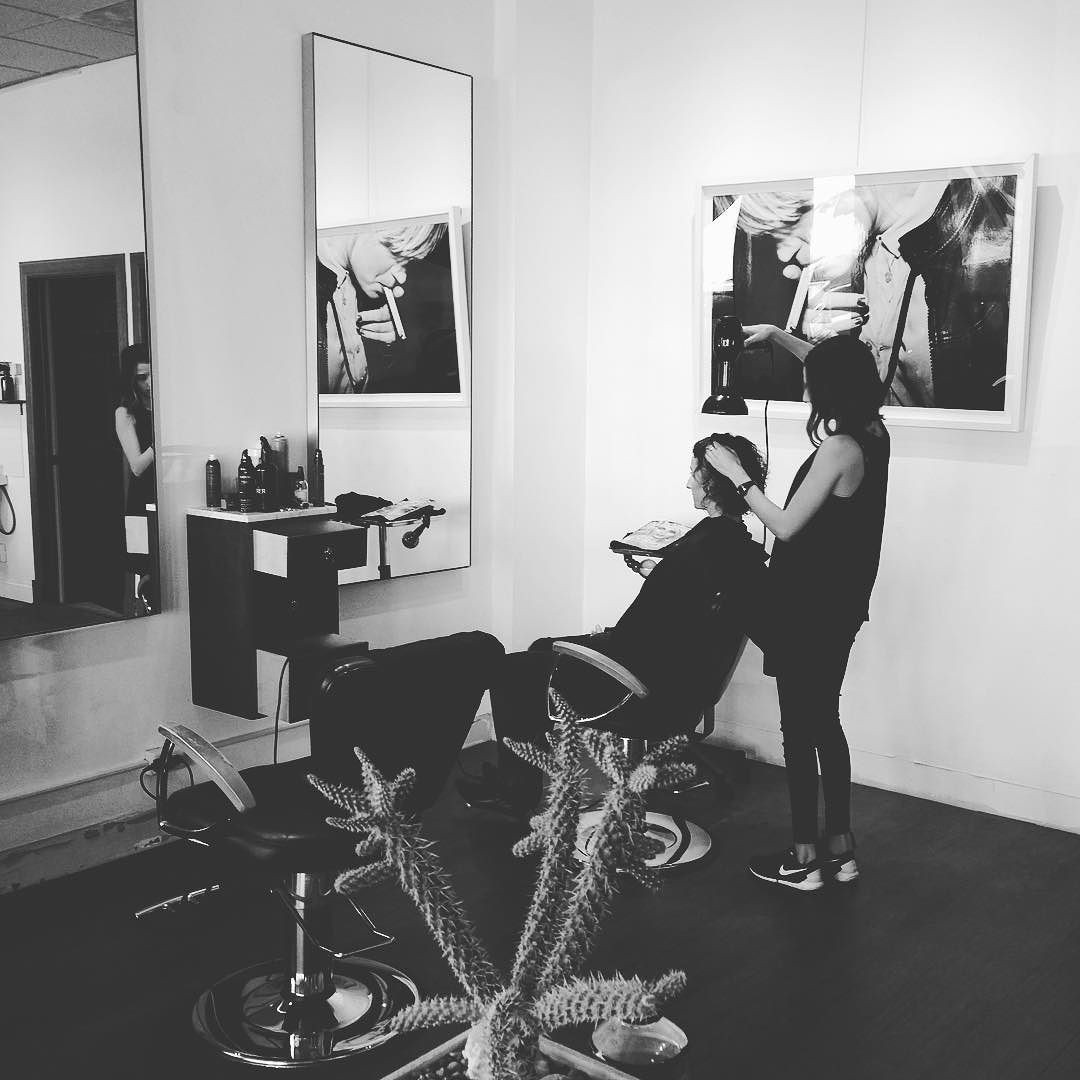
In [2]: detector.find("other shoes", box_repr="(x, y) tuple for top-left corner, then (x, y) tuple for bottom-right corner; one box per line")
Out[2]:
(501, 800), (537, 820)
(481, 759), (506, 800)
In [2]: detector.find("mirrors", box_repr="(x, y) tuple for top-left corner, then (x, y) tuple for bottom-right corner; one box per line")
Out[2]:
(302, 31), (474, 584)
(1, 1), (165, 640)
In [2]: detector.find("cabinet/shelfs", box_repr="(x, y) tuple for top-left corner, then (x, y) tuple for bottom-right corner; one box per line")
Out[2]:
(183, 506), (367, 723)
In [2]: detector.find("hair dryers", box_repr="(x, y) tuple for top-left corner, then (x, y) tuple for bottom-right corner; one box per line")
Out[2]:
(701, 314), (773, 417)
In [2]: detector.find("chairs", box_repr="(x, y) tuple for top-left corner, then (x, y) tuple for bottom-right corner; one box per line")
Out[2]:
(548, 567), (772, 876)
(157, 627), (506, 1070)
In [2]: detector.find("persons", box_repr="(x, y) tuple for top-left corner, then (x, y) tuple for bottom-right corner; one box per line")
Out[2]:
(803, 175), (1017, 413)
(317, 223), (448, 394)
(492, 433), (767, 819)
(712, 190), (807, 403)
(704, 335), (891, 891)
(115, 343), (155, 515)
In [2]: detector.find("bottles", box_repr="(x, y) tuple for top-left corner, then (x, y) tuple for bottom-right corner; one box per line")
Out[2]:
(294, 465), (309, 504)
(238, 449), (256, 512)
(310, 449), (326, 508)
(270, 436), (288, 473)
(206, 454), (222, 507)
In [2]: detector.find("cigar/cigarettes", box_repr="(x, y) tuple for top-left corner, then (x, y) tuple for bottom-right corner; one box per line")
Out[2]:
(383, 287), (407, 339)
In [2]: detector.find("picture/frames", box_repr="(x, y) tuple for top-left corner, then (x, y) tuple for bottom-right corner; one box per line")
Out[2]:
(698, 155), (1037, 433)
(319, 204), (471, 411)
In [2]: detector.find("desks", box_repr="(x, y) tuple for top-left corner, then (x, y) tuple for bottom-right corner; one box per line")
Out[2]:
(611, 548), (664, 561)
(352, 515), (448, 577)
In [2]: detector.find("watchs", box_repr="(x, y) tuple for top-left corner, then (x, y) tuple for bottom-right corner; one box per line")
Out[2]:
(737, 481), (756, 497)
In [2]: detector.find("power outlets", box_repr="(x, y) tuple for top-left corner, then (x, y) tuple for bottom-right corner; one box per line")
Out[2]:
(145, 749), (189, 778)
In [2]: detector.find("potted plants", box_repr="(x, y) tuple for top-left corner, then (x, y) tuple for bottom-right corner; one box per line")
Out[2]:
(306, 686), (704, 1080)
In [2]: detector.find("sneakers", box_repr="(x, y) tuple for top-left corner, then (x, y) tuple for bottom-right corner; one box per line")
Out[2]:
(748, 846), (824, 891)
(818, 850), (861, 883)
